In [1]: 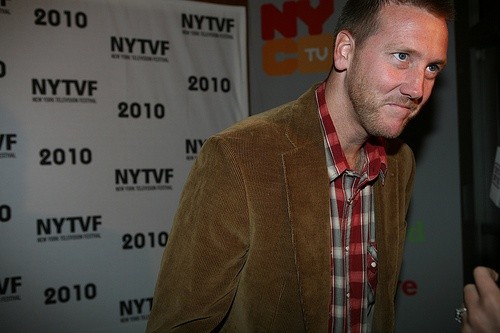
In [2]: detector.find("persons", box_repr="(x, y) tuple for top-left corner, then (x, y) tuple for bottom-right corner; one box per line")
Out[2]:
(457, 265), (500, 333)
(150, 0), (447, 333)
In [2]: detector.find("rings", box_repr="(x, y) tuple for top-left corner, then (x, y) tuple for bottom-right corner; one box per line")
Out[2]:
(455, 307), (467, 323)
